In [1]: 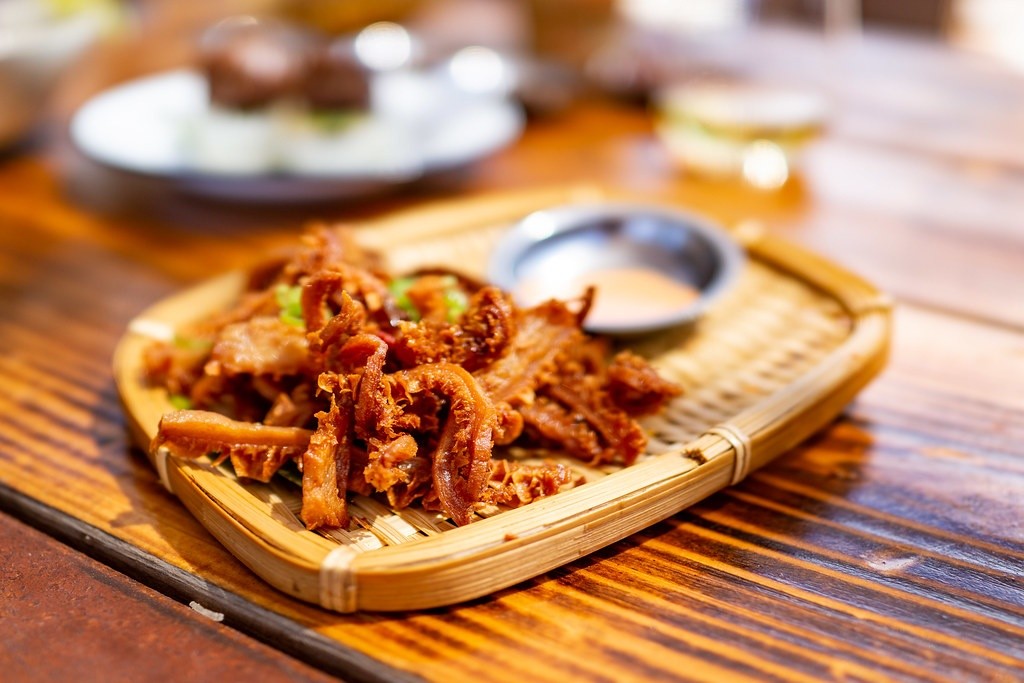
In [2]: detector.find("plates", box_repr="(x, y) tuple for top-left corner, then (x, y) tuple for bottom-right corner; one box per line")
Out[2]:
(110, 182), (891, 608)
(67, 72), (527, 203)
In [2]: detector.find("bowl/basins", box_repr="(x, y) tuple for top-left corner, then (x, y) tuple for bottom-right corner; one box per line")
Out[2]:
(652, 84), (829, 177)
(486, 202), (740, 332)
